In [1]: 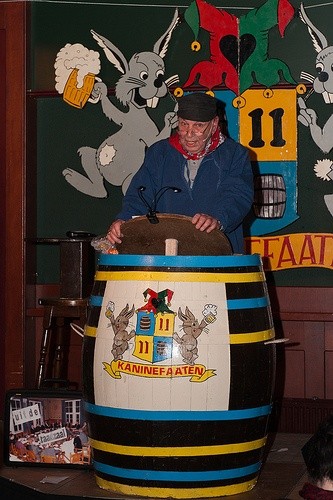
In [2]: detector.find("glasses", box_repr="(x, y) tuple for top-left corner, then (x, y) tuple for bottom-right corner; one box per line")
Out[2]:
(175, 122), (211, 137)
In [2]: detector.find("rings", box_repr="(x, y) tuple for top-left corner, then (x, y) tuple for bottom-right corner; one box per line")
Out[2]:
(108, 230), (111, 235)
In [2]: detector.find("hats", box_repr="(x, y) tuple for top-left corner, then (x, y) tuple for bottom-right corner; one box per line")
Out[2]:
(176, 92), (218, 123)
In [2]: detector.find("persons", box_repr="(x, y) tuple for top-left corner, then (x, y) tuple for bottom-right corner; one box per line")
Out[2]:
(299, 416), (333, 500)
(106, 92), (254, 254)
(10, 420), (89, 461)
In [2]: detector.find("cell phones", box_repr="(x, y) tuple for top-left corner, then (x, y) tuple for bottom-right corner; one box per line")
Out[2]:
(67, 230), (93, 238)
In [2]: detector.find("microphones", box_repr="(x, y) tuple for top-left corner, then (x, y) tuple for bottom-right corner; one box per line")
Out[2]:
(138, 186), (152, 217)
(203, 119), (214, 141)
(150, 187), (180, 215)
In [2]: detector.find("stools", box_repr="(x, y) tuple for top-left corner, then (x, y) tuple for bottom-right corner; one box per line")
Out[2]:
(36, 297), (94, 402)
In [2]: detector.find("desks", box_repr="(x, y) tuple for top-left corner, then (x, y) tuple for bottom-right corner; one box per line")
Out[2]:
(1, 421), (317, 500)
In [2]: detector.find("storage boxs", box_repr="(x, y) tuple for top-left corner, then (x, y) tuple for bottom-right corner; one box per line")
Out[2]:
(3, 388), (91, 469)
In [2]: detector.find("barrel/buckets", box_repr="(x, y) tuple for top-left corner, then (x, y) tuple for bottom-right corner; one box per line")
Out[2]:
(83, 256), (277, 497)
